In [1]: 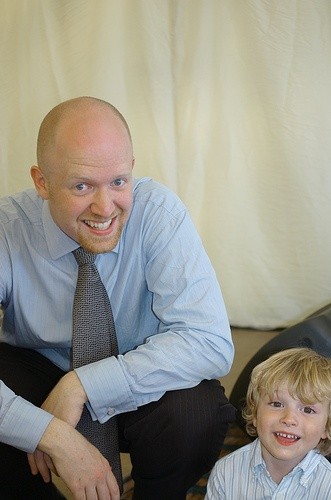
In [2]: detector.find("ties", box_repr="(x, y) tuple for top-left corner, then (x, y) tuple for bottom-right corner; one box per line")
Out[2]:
(71, 247), (124, 495)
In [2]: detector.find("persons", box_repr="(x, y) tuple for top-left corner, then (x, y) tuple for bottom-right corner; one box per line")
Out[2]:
(204, 345), (331, 500)
(0, 95), (237, 500)
